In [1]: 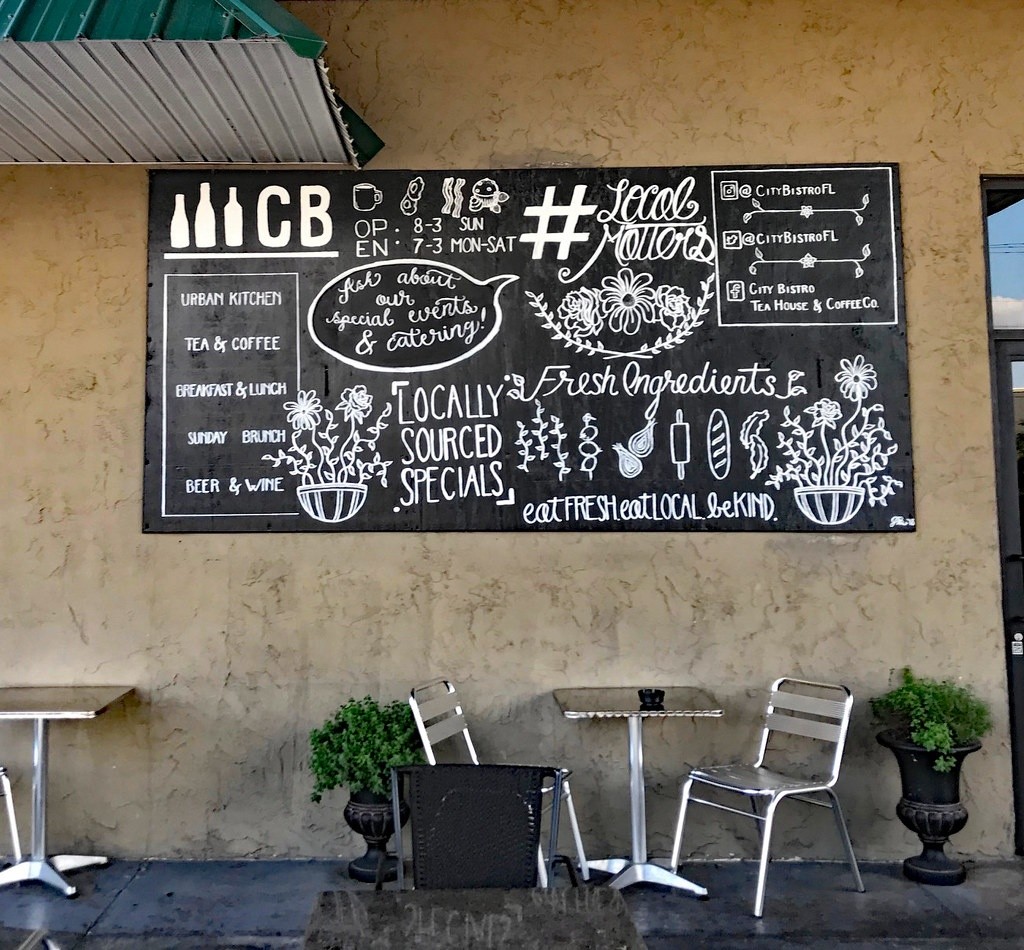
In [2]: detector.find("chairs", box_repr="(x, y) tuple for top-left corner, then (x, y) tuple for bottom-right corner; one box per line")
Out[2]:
(407, 677), (590, 888)
(667, 676), (866, 916)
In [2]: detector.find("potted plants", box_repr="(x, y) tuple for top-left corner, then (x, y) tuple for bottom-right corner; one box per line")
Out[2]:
(305, 694), (423, 882)
(865, 666), (992, 886)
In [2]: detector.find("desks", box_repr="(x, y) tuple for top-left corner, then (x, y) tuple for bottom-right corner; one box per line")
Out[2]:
(0, 680), (136, 898)
(552, 686), (726, 896)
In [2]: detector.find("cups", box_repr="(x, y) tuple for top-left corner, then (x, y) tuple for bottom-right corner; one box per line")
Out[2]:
(638, 689), (665, 706)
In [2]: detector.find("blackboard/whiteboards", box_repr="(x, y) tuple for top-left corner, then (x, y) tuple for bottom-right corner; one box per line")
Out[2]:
(140, 162), (916, 535)
